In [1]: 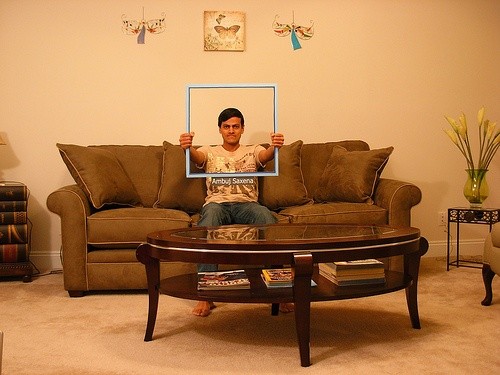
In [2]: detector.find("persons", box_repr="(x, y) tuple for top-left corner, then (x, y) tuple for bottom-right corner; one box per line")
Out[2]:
(178, 108), (294, 317)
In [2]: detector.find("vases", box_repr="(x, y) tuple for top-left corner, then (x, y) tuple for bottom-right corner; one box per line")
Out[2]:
(464, 168), (489, 208)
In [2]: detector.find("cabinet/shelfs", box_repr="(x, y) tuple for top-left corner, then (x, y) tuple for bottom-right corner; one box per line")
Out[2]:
(0, 185), (34, 283)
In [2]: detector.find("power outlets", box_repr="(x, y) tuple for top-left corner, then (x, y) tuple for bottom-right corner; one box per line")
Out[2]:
(437, 212), (446, 225)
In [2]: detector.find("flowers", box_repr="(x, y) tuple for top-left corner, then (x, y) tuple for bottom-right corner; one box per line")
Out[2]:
(442, 107), (500, 198)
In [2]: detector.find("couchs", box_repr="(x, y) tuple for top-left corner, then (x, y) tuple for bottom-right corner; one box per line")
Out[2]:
(47, 140), (422, 299)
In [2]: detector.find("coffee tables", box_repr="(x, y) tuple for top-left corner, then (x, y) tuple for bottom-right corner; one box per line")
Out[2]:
(447, 208), (500, 272)
(135, 222), (429, 367)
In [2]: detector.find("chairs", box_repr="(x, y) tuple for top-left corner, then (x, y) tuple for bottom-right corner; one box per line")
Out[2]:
(481, 221), (500, 306)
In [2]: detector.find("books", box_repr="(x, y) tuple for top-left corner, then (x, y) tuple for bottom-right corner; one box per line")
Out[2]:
(198, 269), (250, 290)
(261, 268), (317, 288)
(319, 259), (386, 286)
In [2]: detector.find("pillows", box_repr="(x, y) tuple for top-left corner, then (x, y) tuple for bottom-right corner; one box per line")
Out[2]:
(152, 141), (208, 214)
(257, 140), (314, 211)
(314, 145), (395, 206)
(56, 142), (144, 210)
